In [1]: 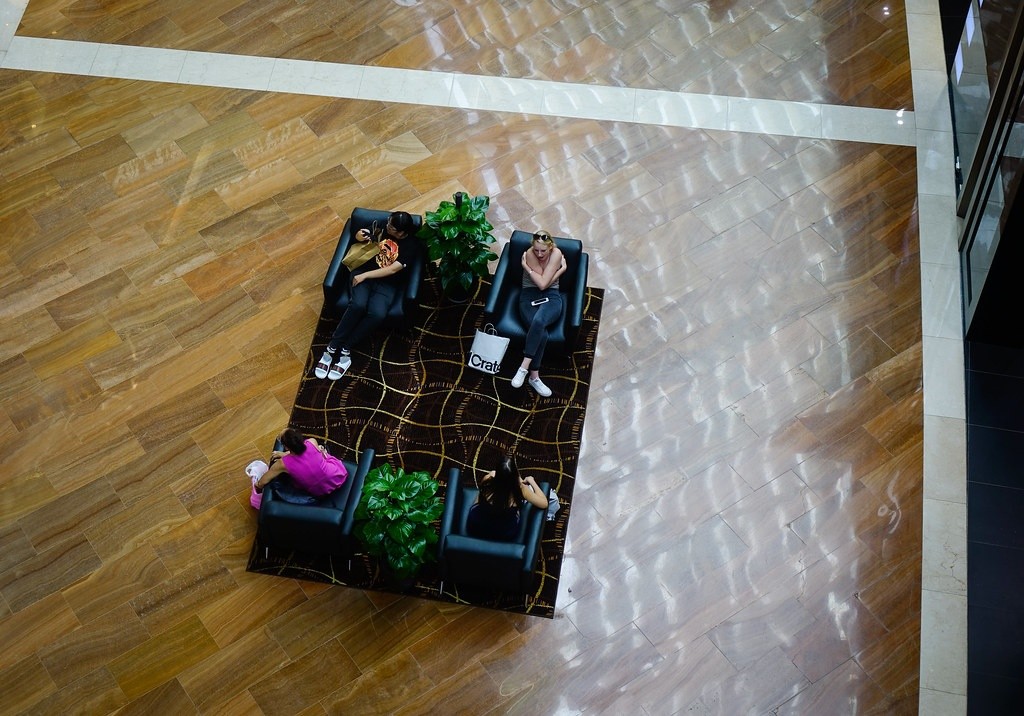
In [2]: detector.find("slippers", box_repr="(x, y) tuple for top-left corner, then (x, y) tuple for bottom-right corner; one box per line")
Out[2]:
(328, 356), (351, 380)
(314, 352), (332, 379)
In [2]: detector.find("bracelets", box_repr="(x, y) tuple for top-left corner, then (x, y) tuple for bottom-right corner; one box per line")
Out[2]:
(527, 269), (534, 276)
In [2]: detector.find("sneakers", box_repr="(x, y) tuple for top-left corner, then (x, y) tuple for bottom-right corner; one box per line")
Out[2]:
(511, 367), (529, 388)
(529, 377), (552, 397)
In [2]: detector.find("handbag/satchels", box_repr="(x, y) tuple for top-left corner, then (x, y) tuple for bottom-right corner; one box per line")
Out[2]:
(467, 323), (510, 374)
(244, 460), (270, 509)
(546, 488), (561, 522)
(341, 239), (380, 273)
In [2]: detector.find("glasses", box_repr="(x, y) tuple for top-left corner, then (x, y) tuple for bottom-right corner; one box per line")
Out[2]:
(533, 234), (553, 243)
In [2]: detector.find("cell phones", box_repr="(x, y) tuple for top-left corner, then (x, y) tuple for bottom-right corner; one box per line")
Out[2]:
(531, 297), (549, 306)
(361, 230), (371, 239)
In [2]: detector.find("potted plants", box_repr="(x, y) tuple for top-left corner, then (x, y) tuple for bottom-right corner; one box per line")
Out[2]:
(354, 462), (444, 586)
(415, 191), (499, 303)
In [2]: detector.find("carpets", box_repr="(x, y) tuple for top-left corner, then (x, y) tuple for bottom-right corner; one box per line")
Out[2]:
(245, 271), (605, 619)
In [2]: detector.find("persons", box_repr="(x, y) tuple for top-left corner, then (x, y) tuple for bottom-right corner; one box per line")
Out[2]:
(466, 451), (548, 543)
(254, 428), (349, 502)
(511, 230), (567, 397)
(323, 210), (417, 364)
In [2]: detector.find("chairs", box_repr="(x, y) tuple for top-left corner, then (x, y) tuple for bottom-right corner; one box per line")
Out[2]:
(484, 230), (589, 348)
(260, 436), (374, 536)
(323, 207), (423, 317)
(439, 468), (550, 574)
